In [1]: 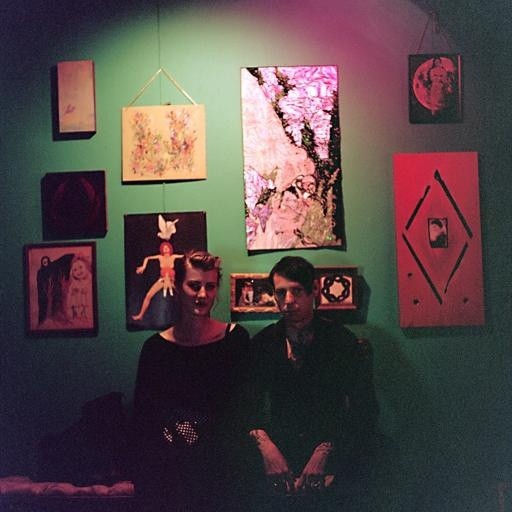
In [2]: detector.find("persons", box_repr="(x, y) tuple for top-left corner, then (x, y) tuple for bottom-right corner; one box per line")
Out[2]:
(426, 56), (451, 116)
(428, 219), (447, 249)
(36, 255), (62, 328)
(133, 243), (186, 322)
(233, 254), (385, 510)
(66, 256), (94, 328)
(130, 248), (253, 510)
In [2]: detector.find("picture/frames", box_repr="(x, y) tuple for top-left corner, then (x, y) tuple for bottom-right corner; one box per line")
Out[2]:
(230, 272), (283, 314)
(23, 241), (99, 336)
(313, 264), (360, 312)
(123, 210), (209, 331)
